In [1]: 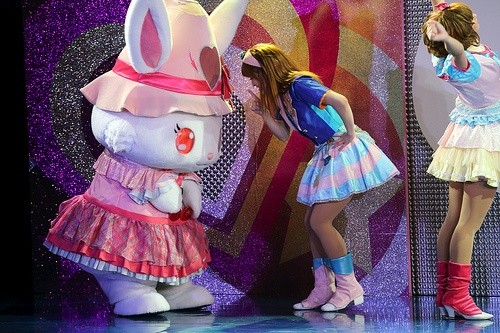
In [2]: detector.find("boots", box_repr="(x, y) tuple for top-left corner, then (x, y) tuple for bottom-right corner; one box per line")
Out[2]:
(293, 256), (337, 309)
(321, 251), (363, 311)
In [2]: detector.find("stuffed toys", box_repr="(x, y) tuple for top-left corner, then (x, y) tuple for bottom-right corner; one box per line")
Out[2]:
(43, 1), (251, 319)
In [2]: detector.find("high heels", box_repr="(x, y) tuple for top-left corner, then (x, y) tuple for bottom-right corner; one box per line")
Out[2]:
(435, 259), (449, 315)
(441, 260), (494, 320)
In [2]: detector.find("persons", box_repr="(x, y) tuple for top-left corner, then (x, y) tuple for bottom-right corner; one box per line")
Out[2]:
(420, 0), (500, 324)
(242, 43), (401, 313)
